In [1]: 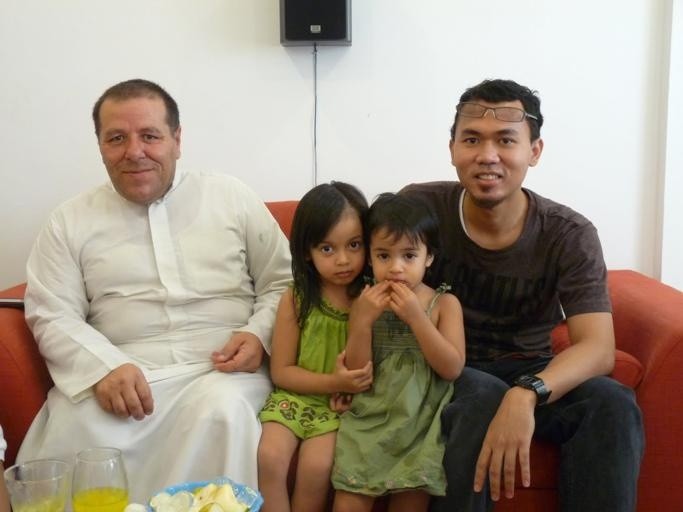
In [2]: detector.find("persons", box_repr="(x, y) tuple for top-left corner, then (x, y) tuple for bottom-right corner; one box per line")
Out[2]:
(0, 422), (12, 512)
(330, 192), (466, 511)
(257, 181), (374, 511)
(14, 80), (293, 504)
(395, 79), (645, 512)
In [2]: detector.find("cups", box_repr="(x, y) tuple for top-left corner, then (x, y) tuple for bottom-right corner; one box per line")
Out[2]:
(2, 446), (130, 512)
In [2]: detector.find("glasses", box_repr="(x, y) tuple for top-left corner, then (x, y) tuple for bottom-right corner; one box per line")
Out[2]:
(452, 99), (541, 125)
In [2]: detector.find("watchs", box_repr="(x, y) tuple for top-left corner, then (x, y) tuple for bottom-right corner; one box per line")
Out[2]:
(515, 375), (551, 406)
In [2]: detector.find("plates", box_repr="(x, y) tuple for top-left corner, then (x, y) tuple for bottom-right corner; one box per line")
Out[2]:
(146, 476), (262, 512)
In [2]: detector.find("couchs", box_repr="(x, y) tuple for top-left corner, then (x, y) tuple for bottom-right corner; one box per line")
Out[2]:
(0, 199), (681, 512)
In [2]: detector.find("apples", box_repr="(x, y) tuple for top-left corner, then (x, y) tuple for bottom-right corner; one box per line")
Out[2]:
(150, 482), (248, 512)
